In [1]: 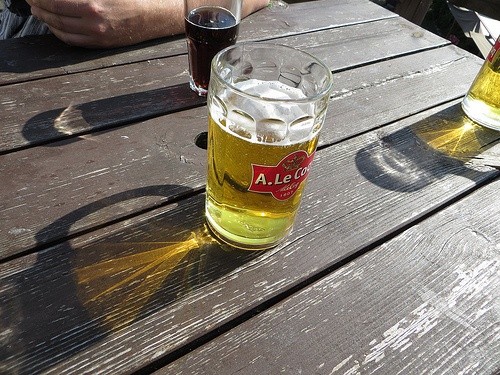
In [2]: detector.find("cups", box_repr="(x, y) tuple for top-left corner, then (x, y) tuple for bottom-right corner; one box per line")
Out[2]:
(461, 34), (500, 132)
(202, 43), (333, 251)
(182, 0), (241, 97)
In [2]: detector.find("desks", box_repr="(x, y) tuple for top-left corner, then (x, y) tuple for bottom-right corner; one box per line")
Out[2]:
(0, 0), (500, 375)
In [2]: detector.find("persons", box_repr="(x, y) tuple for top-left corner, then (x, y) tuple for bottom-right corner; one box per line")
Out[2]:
(0, 0), (270, 47)
